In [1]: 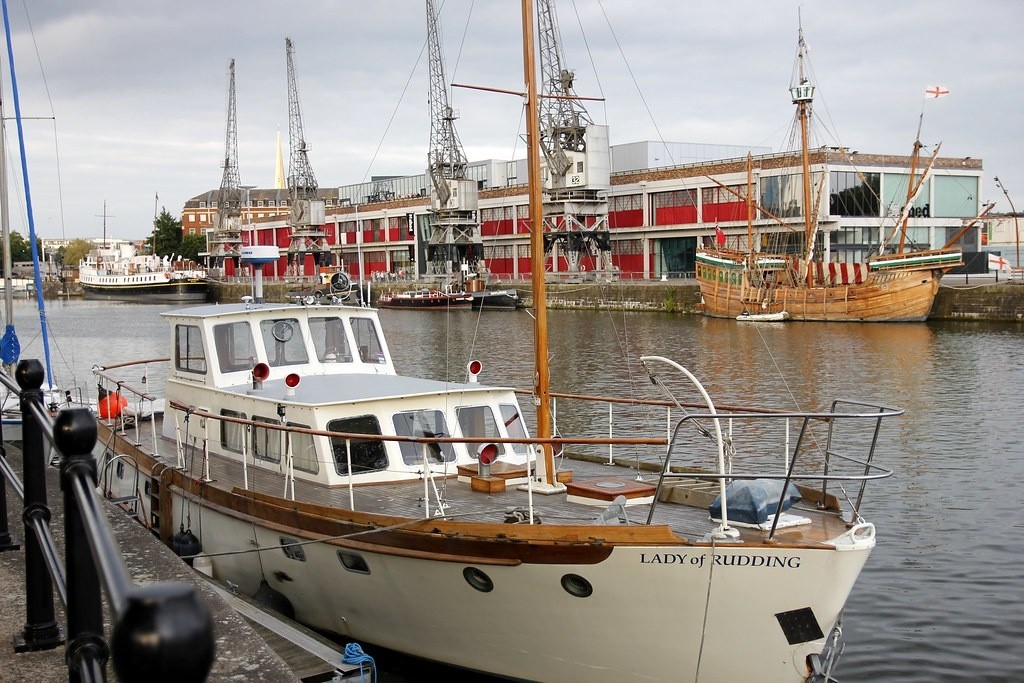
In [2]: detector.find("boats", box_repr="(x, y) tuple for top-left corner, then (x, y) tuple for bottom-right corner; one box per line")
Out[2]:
(377, 284), (476, 313)
(418, 275), (520, 312)
(285, 282), (365, 309)
(73, 240), (209, 303)
(0, 0), (86, 442)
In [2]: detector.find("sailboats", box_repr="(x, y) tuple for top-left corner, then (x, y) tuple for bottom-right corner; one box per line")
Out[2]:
(693, 1), (998, 321)
(96, 0), (911, 683)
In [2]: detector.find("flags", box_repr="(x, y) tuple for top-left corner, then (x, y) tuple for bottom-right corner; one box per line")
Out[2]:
(924, 86), (949, 100)
(987, 253), (1011, 271)
(715, 226), (726, 247)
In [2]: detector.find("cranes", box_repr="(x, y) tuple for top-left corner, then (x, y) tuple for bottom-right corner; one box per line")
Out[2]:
(418, 0), (492, 279)
(276, 37), (337, 279)
(529, 0), (618, 286)
(201, 46), (250, 285)
